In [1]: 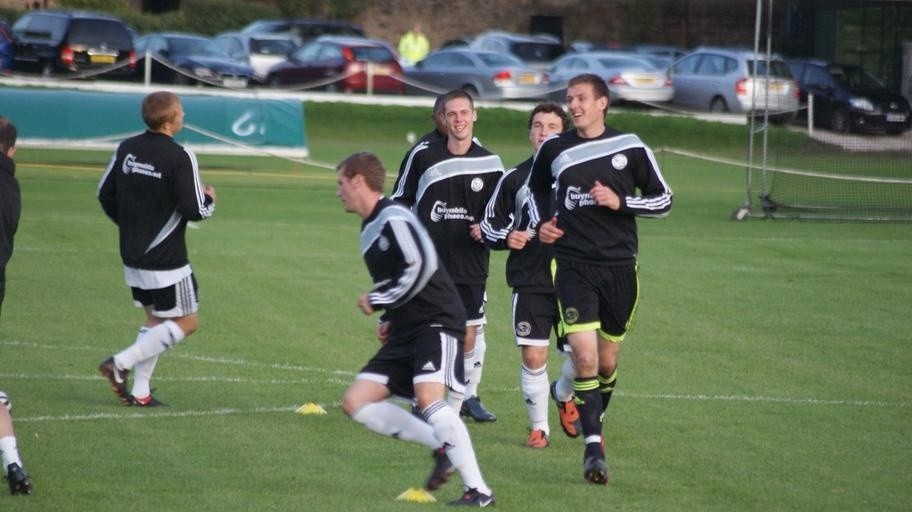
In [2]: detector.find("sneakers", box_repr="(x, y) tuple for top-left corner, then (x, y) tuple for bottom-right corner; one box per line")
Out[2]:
(459, 396), (497, 422)
(6, 461), (33, 495)
(550, 380), (582, 438)
(582, 454), (607, 484)
(123, 391), (168, 407)
(99, 354), (134, 406)
(525, 429), (550, 448)
(444, 485), (497, 508)
(425, 446), (457, 491)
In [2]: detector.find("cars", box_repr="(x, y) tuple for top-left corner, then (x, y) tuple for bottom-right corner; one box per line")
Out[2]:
(786, 60), (910, 135)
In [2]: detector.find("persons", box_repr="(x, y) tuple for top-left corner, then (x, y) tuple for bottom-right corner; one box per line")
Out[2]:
(337, 152), (496, 506)
(0, 392), (34, 498)
(479, 103), (582, 446)
(2, 116), (22, 304)
(389, 87), (513, 421)
(528, 74), (673, 484)
(98, 91), (216, 405)
